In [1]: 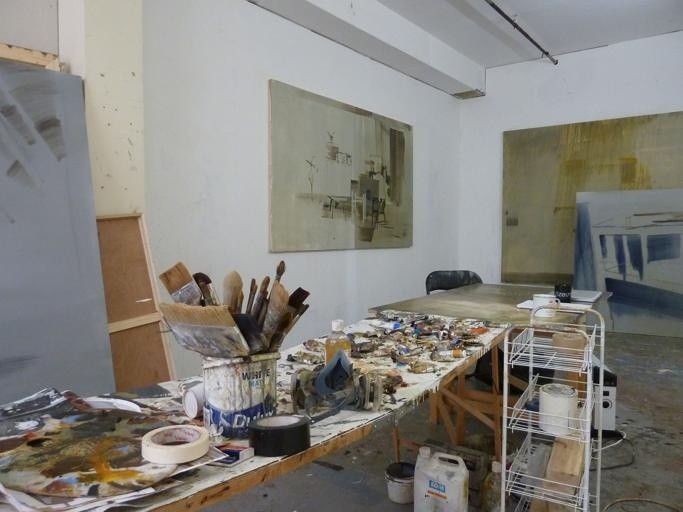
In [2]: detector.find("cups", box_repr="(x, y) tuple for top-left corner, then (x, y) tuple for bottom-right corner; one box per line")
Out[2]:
(554, 284), (573, 303)
(181, 381), (203, 420)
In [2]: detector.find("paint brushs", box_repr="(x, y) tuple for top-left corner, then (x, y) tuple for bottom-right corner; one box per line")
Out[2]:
(223, 260), (310, 356)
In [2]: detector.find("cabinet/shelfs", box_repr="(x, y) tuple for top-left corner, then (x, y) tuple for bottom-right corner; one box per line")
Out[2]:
(498, 298), (608, 512)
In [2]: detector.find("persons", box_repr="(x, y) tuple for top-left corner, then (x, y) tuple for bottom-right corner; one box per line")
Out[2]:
(375, 165), (390, 225)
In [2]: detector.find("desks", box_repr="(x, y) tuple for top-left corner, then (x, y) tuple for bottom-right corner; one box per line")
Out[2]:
(364, 281), (617, 465)
(1, 307), (512, 511)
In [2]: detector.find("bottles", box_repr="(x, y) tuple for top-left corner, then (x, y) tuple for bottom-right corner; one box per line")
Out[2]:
(323, 318), (353, 366)
(482, 459), (504, 512)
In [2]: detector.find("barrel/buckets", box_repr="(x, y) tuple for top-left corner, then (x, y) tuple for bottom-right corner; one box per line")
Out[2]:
(537, 382), (579, 435)
(200, 352), (282, 441)
(555, 281), (572, 303)
(412, 446), (471, 512)
(384, 461), (415, 505)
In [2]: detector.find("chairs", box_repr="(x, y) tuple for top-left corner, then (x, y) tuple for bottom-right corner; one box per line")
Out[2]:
(422, 267), (484, 296)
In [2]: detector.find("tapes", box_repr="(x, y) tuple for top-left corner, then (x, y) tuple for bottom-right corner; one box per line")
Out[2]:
(248, 414), (310, 457)
(141, 424), (210, 465)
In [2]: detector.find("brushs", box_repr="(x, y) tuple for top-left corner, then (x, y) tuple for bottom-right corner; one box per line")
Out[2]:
(157, 261), (269, 359)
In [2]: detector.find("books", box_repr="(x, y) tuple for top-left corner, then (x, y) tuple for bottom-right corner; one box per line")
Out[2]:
(568, 288), (603, 303)
(517, 298), (592, 315)
